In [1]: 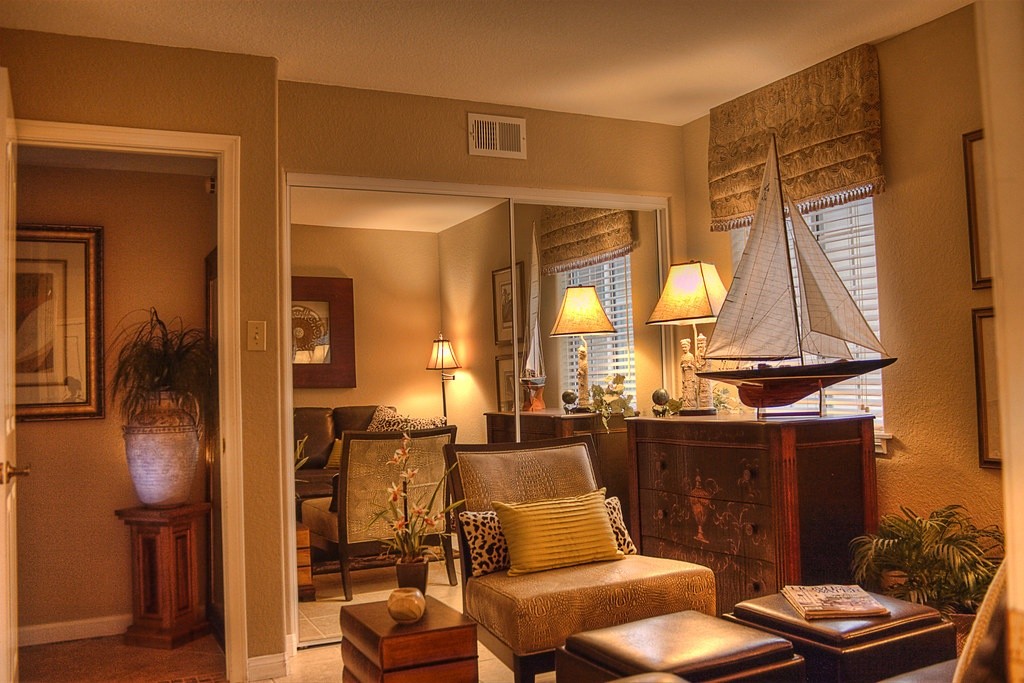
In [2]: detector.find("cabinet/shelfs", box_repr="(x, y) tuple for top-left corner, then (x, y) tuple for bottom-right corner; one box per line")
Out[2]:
(623, 414), (878, 620)
(484, 408), (640, 538)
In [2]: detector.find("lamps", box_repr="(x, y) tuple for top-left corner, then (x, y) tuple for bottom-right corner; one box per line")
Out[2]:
(426, 330), (462, 426)
(550, 284), (617, 414)
(646, 259), (727, 417)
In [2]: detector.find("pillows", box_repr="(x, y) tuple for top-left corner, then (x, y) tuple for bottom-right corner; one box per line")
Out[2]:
(372, 417), (447, 430)
(368, 405), (396, 431)
(491, 487), (624, 577)
(459, 497), (638, 579)
(324, 438), (343, 470)
(329, 473), (339, 513)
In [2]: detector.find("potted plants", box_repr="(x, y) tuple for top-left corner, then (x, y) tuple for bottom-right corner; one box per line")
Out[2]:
(100, 306), (219, 511)
(850, 504), (1006, 659)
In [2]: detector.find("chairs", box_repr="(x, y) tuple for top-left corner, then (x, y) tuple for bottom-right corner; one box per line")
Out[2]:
(443, 433), (716, 683)
(302, 425), (458, 601)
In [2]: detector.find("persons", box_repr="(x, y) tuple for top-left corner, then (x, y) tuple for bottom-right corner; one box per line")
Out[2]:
(680, 339), (696, 407)
(695, 332), (713, 407)
(578, 345), (589, 405)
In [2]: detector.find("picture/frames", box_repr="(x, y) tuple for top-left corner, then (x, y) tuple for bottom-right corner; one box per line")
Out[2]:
(491, 261), (524, 345)
(963, 127), (994, 290)
(495, 351), (532, 412)
(15, 222), (105, 423)
(291, 275), (357, 388)
(971, 306), (1002, 469)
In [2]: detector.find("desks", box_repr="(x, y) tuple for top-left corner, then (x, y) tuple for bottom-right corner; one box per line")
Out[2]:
(340, 595), (479, 683)
(296, 522), (317, 603)
(116, 502), (214, 650)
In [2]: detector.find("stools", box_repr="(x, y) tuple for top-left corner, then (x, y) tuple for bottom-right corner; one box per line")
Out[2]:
(723, 583), (957, 683)
(556, 609), (809, 683)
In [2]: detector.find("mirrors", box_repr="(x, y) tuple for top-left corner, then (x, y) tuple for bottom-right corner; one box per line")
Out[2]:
(288, 184), (665, 649)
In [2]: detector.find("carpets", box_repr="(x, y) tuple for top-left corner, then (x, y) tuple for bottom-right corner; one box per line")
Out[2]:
(313, 547), (462, 575)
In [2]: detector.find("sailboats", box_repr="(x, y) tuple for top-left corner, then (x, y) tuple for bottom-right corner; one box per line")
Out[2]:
(694, 132), (900, 409)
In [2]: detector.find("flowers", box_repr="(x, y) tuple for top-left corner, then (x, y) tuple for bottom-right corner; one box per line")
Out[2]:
(368, 427), (468, 563)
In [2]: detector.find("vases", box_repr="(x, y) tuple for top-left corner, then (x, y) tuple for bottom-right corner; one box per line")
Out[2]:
(397, 558), (430, 597)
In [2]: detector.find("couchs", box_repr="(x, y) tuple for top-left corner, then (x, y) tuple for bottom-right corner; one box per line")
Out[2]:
(293, 405), (396, 520)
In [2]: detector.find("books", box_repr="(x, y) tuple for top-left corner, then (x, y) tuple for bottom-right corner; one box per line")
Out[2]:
(780, 584), (891, 619)
